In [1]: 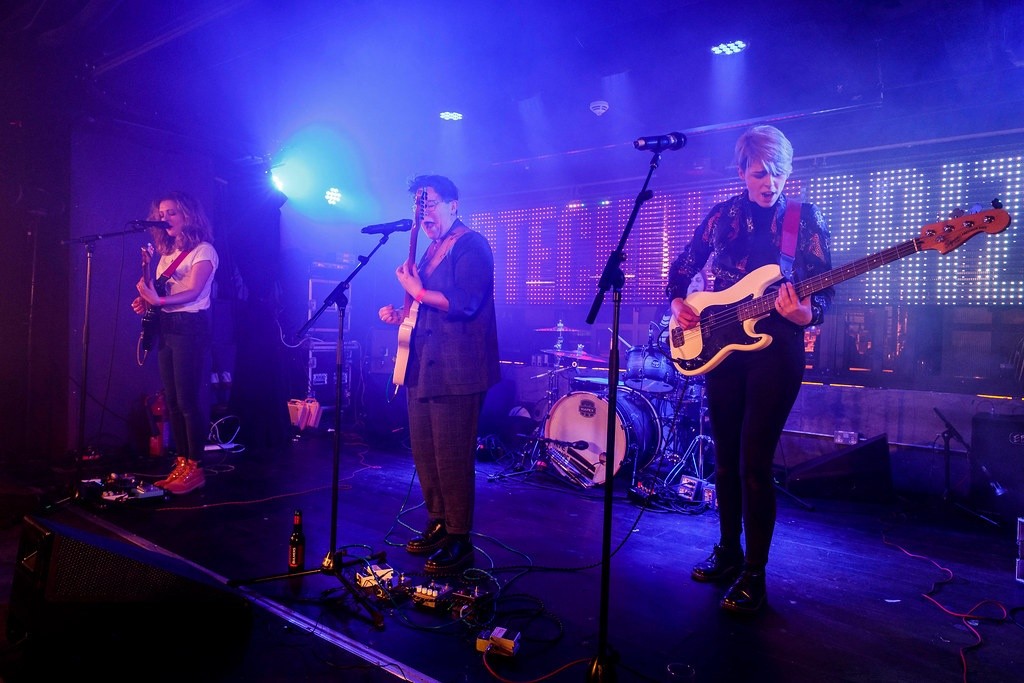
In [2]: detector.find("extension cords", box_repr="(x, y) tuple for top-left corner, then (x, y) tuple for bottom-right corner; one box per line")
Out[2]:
(204, 445), (235, 450)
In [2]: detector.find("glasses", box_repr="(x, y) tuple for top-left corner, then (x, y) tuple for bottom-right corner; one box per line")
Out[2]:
(412, 199), (453, 215)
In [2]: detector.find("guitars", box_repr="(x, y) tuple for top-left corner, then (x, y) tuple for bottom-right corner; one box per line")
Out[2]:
(139, 243), (165, 352)
(392, 187), (429, 386)
(668, 197), (1012, 377)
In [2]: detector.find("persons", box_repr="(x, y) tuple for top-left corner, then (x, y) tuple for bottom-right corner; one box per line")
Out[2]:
(665, 126), (834, 610)
(131, 191), (218, 494)
(650, 273), (706, 462)
(377, 176), (499, 573)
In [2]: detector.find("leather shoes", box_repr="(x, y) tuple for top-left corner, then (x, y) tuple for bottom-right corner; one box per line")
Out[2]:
(407, 519), (447, 552)
(721, 571), (765, 609)
(425, 537), (473, 571)
(692, 543), (744, 580)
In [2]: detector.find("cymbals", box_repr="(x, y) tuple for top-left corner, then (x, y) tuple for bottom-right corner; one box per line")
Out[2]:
(534, 326), (581, 332)
(539, 349), (609, 364)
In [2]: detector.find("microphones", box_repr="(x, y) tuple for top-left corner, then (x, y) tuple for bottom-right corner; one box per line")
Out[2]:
(572, 441), (589, 449)
(361, 219), (414, 235)
(649, 324), (654, 341)
(634, 132), (687, 151)
(989, 479), (1008, 496)
(130, 219), (170, 230)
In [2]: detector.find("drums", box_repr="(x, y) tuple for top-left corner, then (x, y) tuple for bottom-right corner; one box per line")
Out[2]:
(545, 385), (662, 489)
(622, 344), (677, 395)
(570, 375), (626, 397)
(654, 369), (702, 406)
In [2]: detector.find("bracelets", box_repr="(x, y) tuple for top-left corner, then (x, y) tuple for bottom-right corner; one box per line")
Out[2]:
(416, 288), (426, 303)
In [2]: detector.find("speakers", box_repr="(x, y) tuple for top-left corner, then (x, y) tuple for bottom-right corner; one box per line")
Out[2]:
(7, 515), (254, 683)
(969, 410), (1024, 519)
(787, 432), (894, 505)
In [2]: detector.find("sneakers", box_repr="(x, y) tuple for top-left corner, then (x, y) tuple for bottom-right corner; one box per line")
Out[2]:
(154, 456), (189, 488)
(164, 459), (205, 494)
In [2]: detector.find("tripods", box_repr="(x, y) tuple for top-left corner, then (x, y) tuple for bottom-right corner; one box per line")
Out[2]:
(232, 236), (391, 630)
(892, 407), (1001, 528)
(658, 388), (716, 496)
(42, 227), (142, 512)
(487, 433), (583, 491)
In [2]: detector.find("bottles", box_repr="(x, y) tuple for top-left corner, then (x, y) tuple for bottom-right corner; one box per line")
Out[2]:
(288, 510), (304, 571)
